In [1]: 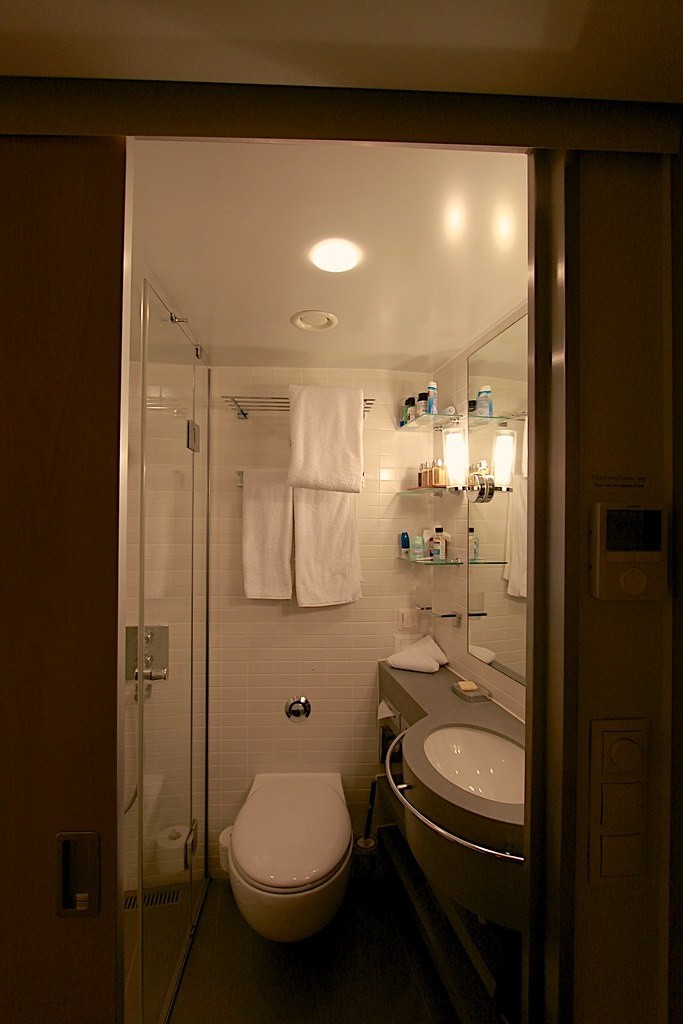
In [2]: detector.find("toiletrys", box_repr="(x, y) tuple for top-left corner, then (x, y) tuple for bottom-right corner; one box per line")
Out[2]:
(468, 526), (476, 561)
(410, 536), (425, 560)
(429, 526), (448, 559)
(476, 536), (480, 559)
(399, 530), (410, 558)
(417, 458), (447, 486)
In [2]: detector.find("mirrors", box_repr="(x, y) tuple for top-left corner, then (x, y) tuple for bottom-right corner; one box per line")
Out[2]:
(467, 313), (527, 685)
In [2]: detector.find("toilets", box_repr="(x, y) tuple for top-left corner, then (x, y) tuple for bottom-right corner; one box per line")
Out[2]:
(229, 773), (354, 943)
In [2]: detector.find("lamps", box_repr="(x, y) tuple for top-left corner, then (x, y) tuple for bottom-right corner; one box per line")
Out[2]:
(441, 428), (466, 495)
(495, 431), (516, 498)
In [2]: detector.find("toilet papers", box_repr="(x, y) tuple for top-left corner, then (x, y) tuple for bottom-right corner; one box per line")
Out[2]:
(155, 826), (190, 874)
(219, 825), (232, 873)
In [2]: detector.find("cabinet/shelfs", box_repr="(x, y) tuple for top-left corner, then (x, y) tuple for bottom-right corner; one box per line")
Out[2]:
(395, 412), (464, 565)
(374, 774), (524, 1023)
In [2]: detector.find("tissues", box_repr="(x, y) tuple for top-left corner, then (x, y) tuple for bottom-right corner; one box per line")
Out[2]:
(376, 688), (400, 733)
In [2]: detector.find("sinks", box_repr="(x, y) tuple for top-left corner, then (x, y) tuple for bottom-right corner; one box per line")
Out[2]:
(423, 722), (525, 805)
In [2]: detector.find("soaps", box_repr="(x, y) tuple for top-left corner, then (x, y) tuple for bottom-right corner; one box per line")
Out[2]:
(458, 680), (478, 691)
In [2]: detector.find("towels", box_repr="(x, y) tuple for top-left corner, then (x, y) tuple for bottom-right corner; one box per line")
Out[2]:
(241, 465), (292, 601)
(287, 383), (365, 494)
(468, 644), (496, 665)
(503, 473), (527, 598)
(384, 648), (440, 673)
(293, 487), (364, 608)
(402, 635), (449, 666)
